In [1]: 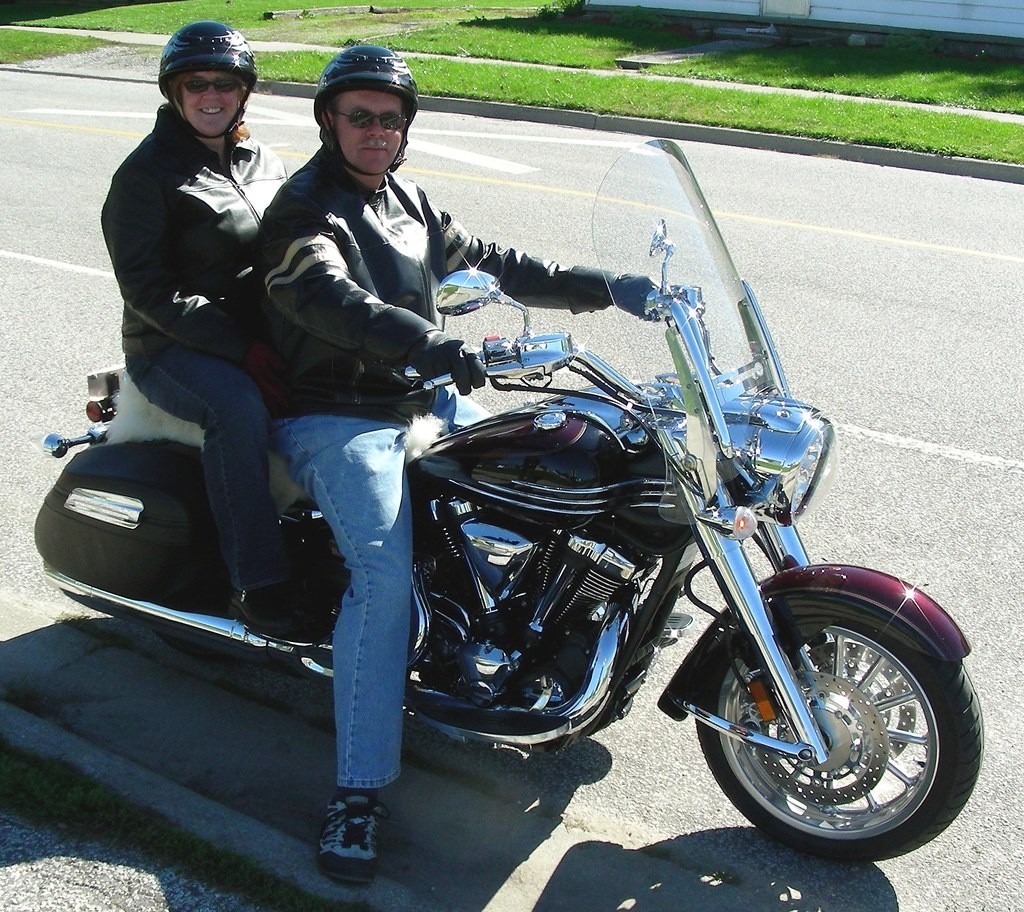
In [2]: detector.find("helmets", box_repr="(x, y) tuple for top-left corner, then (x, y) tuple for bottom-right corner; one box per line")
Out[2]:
(157, 22), (257, 98)
(313, 46), (419, 130)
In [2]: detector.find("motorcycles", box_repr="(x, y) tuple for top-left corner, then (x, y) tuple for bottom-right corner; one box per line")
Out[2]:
(34, 136), (984, 868)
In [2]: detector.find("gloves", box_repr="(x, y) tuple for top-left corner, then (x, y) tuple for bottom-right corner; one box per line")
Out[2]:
(567, 265), (660, 323)
(364, 307), (486, 396)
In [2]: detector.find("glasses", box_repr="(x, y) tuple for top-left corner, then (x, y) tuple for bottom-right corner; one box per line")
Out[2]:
(333, 110), (408, 130)
(176, 77), (240, 94)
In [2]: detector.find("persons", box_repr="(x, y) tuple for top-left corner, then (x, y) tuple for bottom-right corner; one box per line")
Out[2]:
(102, 24), (333, 645)
(244, 44), (655, 882)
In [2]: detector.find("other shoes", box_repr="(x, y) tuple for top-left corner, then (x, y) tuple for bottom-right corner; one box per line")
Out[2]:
(227, 592), (308, 640)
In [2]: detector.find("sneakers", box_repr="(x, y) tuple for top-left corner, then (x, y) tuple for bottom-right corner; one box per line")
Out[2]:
(318, 795), (390, 882)
